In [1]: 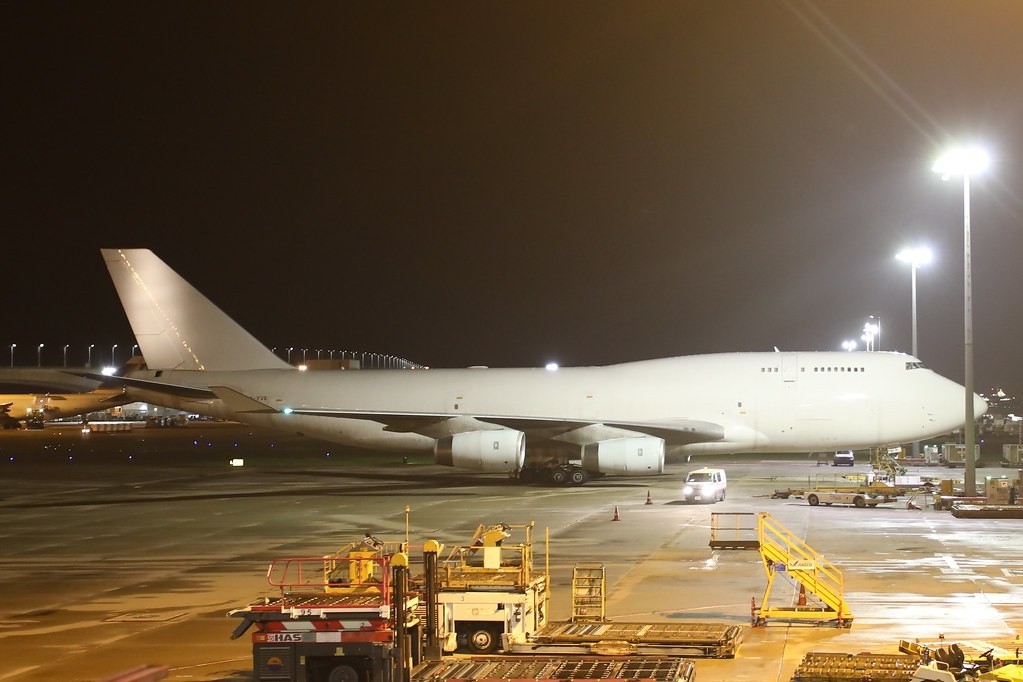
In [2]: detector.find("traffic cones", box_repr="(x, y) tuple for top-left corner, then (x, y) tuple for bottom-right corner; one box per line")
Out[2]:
(644, 490), (653, 505)
(610, 505), (621, 521)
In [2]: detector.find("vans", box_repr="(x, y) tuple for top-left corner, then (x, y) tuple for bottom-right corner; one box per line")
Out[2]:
(683, 467), (727, 505)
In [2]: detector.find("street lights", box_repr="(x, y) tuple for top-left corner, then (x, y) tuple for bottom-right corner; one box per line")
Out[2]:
(895, 247), (934, 358)
(88, 344), (95, 366)
(10, 344), (17, 368)
(63, 344), (70, 367)
(932, 147), (991, 499)
(284, 347), (424, 370)
(132, 344), (138, 357)
(112, 344), (118, 367)
(842, 313), (883, 352)
(36, 343), (45, 368)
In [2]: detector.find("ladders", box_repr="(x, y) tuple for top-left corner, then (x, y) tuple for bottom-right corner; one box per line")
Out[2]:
(754, 511), (856, 621)
(572, 560), (606, 622)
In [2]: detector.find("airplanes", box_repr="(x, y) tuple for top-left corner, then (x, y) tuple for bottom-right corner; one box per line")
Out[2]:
(0, 246), (993, 487)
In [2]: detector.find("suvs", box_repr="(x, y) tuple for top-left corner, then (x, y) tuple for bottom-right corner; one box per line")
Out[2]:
(833, 450), (855, 467)
